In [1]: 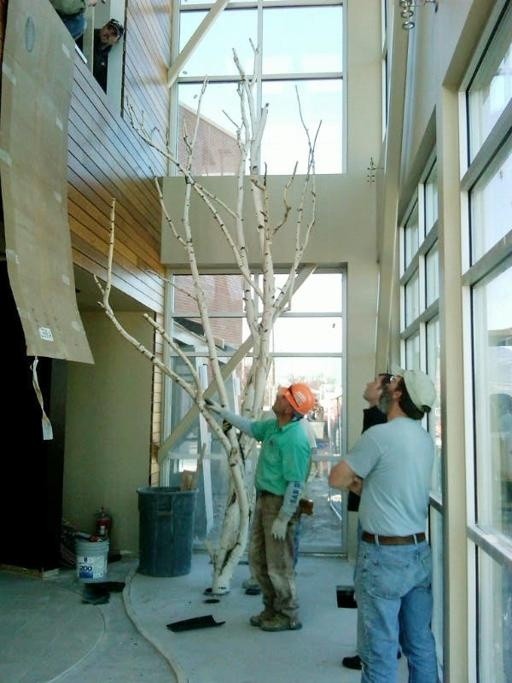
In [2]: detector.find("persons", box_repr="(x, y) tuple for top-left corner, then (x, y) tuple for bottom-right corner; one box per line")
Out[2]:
(342, 373), (402, 670)
(206, 383), (315, 631)
(51, 0), (106, 43)
(75, 18), (124, 94)
(329, 361), (440, 683)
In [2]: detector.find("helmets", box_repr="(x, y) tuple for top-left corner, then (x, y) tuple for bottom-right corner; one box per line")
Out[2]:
(277, 382), (314, 416)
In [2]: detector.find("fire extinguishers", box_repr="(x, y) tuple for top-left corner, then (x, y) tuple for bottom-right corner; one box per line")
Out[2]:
(95, 507), (112, 537)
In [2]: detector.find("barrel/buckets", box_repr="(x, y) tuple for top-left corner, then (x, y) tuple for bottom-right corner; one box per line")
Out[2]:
(73, 537), (109, 584)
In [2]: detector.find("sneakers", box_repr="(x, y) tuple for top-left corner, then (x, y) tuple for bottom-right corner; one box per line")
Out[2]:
(342, 653), (364, 669)
(249, 606), (303, 631)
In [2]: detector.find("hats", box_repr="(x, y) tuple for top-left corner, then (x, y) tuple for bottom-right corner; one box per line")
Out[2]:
(390, 361), (437, 414)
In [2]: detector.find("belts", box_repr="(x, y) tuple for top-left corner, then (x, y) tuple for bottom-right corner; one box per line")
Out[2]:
(360, 530), (427, 545)
(257, 489), (272, 495)
